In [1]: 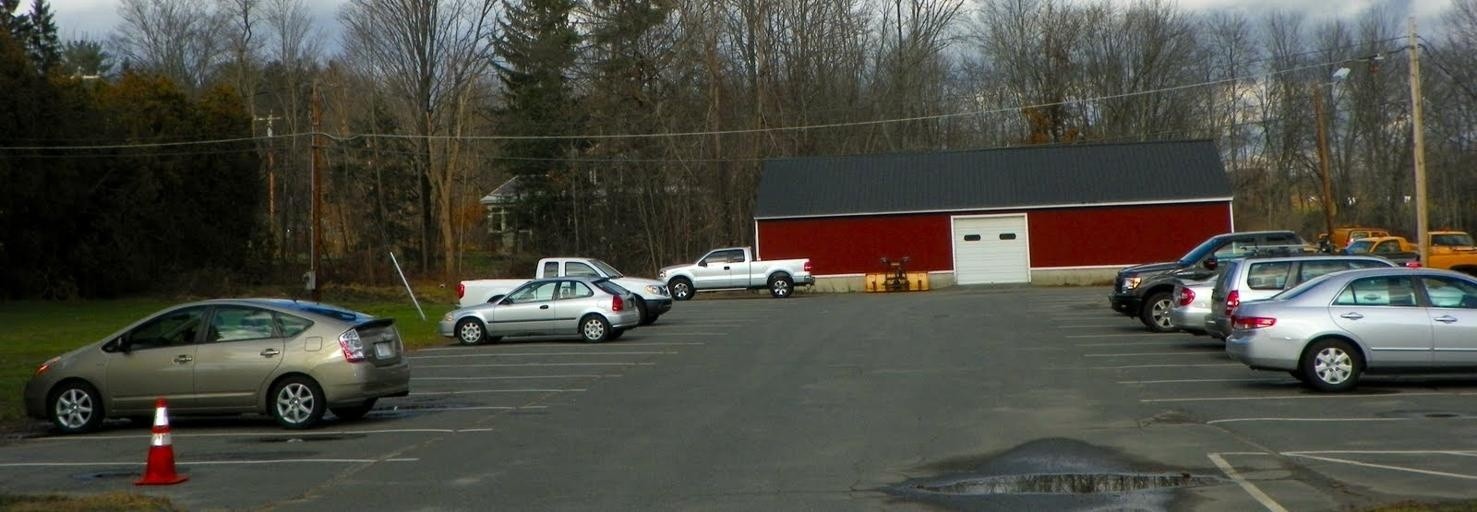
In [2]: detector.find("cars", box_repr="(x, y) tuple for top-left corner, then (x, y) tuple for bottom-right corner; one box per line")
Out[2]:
(435, 256), (672, 344)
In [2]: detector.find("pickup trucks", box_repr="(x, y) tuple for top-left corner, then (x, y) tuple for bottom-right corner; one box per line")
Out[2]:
(656, 246), (815, 298)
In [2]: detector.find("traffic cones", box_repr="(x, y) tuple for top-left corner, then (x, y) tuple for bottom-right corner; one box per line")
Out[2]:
(136, 398), (189, 488)
